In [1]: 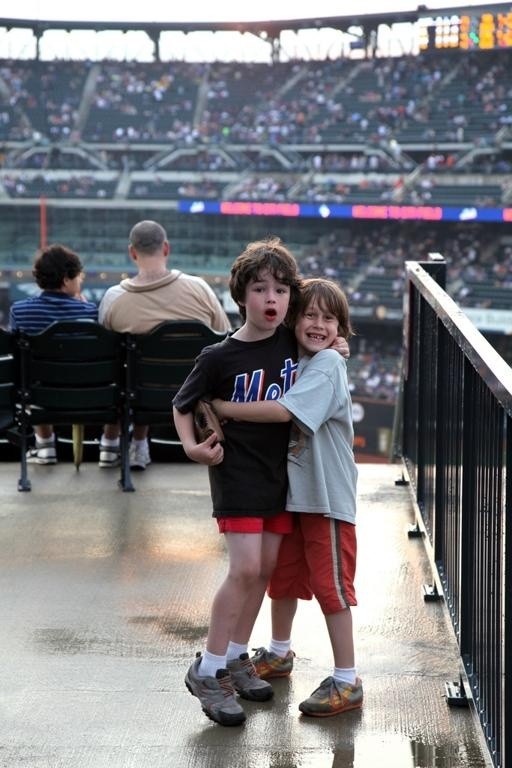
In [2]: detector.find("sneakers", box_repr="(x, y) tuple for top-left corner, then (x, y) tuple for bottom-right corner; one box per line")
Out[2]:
(250, 647), (293, 679)
(227, 653), (274, 702)
(25, 446), (57, 464)
(299, 676), (363, 716)
(129, 445), (151, 469)
(184, 656), (246, 727)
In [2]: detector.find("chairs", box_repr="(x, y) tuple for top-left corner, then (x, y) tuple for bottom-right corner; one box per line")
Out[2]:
(20, 319), (125, 488)
(0, 327), (20, 488)
(123, 318), (228, 489)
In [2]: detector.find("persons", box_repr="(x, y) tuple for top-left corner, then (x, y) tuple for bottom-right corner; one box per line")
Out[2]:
(95, 221), (232, 470)
(208, 279), (368, 720)
(2, 49), (512, 206)
(350, 339), (402, 397)
(1, 213), (512, 308)
(168, 239), (351, 727)
(8, 244), (119, 467)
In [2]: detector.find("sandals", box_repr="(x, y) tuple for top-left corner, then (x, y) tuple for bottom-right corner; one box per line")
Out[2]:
(99, 445), (122, 467)
